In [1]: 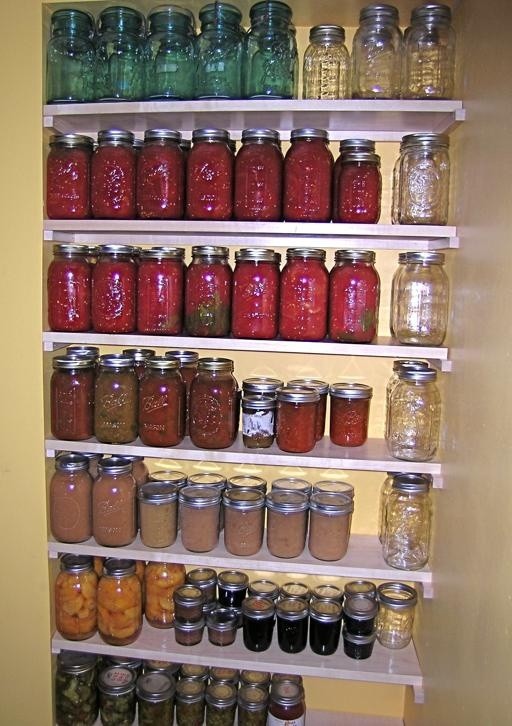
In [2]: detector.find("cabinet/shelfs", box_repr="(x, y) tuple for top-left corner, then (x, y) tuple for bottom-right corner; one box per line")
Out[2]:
(43, 99), (467, 725)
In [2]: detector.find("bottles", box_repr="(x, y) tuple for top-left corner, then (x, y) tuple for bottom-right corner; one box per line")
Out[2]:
(376, 128), (452, 571)
(375, 581), (418, 649)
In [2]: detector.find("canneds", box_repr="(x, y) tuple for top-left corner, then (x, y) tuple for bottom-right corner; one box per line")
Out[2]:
(45, 1), (299, 104)
